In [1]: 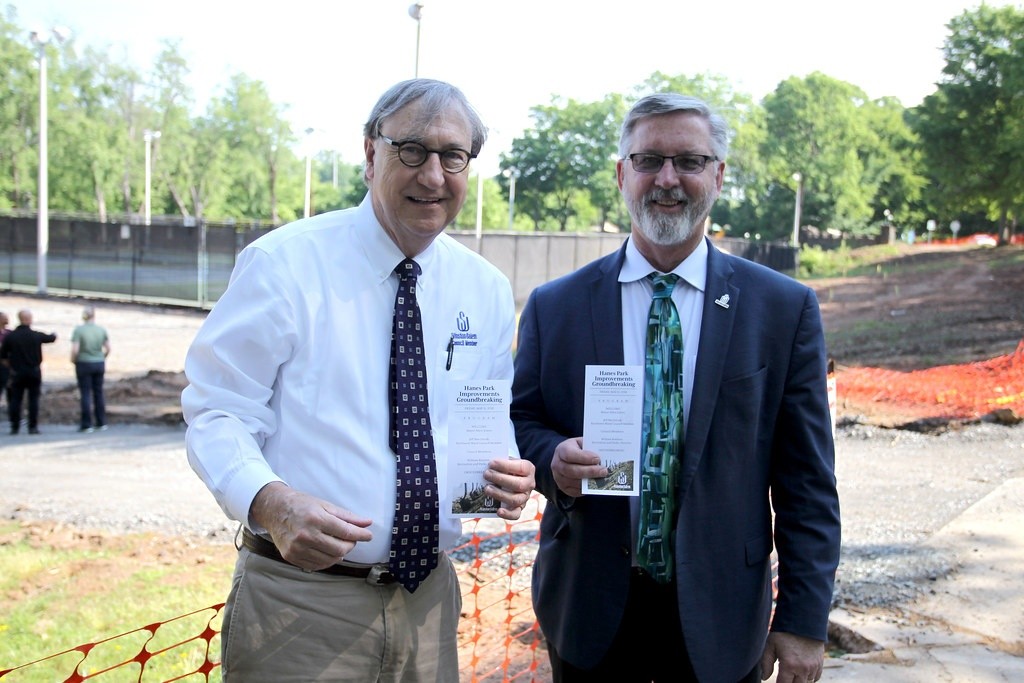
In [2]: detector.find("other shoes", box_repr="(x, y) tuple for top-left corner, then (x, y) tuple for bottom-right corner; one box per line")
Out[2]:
(94, 424), (108, 430)
(76, 426), (94, 433)
(10, 429), (18, 433)
(29, 427), (40, 434)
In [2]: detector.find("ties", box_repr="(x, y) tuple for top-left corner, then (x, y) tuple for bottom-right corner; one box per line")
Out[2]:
(630, 273), (685, 583)
(387, 257), (440, 594)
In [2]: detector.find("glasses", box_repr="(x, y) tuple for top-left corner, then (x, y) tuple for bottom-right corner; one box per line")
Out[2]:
(622, 152), (717, 174)
(379, 132), (478, 174)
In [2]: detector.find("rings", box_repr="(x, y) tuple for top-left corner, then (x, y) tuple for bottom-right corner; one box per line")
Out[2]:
(808, 678), (815, 683)
(518, 504), (525, 511)
(525, 491), (530, 500)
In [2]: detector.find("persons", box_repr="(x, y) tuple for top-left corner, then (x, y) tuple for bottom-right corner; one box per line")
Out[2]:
(0, 308), (58, 435)
(69, 306), (110, 432)
(178, 80), (536, 683)
(508, 93), (842, 683)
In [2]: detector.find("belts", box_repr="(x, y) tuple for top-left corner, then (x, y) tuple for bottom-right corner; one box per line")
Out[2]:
(619, 545), (653, 577)
(241, 525), (444, 586)
